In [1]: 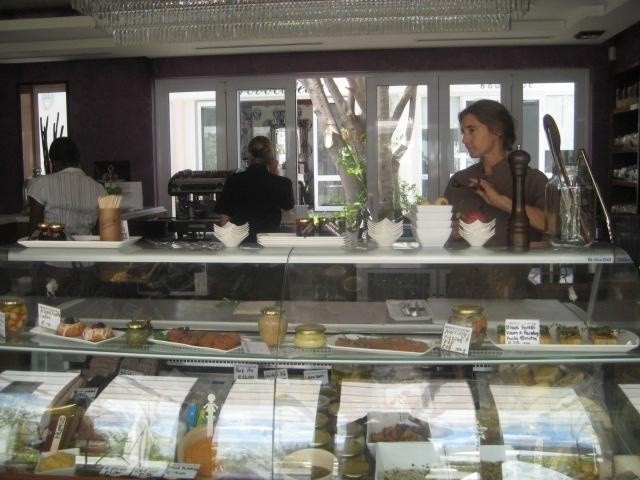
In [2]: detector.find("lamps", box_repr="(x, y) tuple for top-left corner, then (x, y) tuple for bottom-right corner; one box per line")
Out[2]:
(70, 0), (532, 50)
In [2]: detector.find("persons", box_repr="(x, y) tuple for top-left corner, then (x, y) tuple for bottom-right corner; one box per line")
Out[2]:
(27, 136), (109, 298)
(210, 135), (295, 301)
(443, 99), (561, 301)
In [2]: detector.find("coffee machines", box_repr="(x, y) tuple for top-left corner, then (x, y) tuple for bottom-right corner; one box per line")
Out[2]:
(166, 170), (237, 238)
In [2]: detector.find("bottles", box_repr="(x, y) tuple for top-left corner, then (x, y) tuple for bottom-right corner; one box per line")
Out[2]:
(31, 221), (66, 238)
(546, 164), (598, 249)
(295, 215), (348, 237)
(20, 166), (47, 216)
(508, 145), (529, 248)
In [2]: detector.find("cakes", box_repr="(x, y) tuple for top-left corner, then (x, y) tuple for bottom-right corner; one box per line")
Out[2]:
(497, 326), (617, 345)
(57, 317), (86, 336)
(81, 323), (113, 341)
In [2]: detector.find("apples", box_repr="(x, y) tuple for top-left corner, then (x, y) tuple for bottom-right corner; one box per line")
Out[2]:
(466, 210), (486, 224)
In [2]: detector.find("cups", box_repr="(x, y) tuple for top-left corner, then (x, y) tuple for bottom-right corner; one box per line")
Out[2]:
(98, 207), (123, 238)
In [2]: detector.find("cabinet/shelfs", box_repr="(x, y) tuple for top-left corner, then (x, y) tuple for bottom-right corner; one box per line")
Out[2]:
(0, 233), (640, 479)
(606, 60), (640, 273)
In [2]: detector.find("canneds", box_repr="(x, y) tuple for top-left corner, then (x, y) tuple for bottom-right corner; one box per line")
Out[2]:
(126, 321), (147, 346)
(319, 218), (328, 232)
(296, 219), (315, 236)
(294, 324), (326, 348)
(47, 224), (66, 240)
(449, 305), (487, 346)
(0, 298), (29, 339)
(258, 307), (288, 347)
(29, 223), (48, 240)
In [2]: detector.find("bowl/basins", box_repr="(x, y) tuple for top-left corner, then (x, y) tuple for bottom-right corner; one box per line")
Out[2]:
(411, 205), (455, 247)
(363, 409), (520, 480)
(34, 447), (83, 477)
(176, 425), (340, 479)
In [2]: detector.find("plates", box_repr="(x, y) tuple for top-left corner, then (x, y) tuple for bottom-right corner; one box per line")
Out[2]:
(320, 332), (435, 355)
(384, 297), (434, 322)
(366, 217), (405, 247)
(212, 220), (250, 248)
(458, 219), (497, 247)
(17, 234), (142, 249)
(29, 321), (127, 345)
(485, 324), (640, 352)
(256, 233), (354, 248)
(151, 327), (246, 354)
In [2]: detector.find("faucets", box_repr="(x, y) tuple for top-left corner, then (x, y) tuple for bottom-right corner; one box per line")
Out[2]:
(577, 146), (616, 247)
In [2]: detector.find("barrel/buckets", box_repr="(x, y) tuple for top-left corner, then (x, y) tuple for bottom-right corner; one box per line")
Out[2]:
(280, 447), (339, 480)
